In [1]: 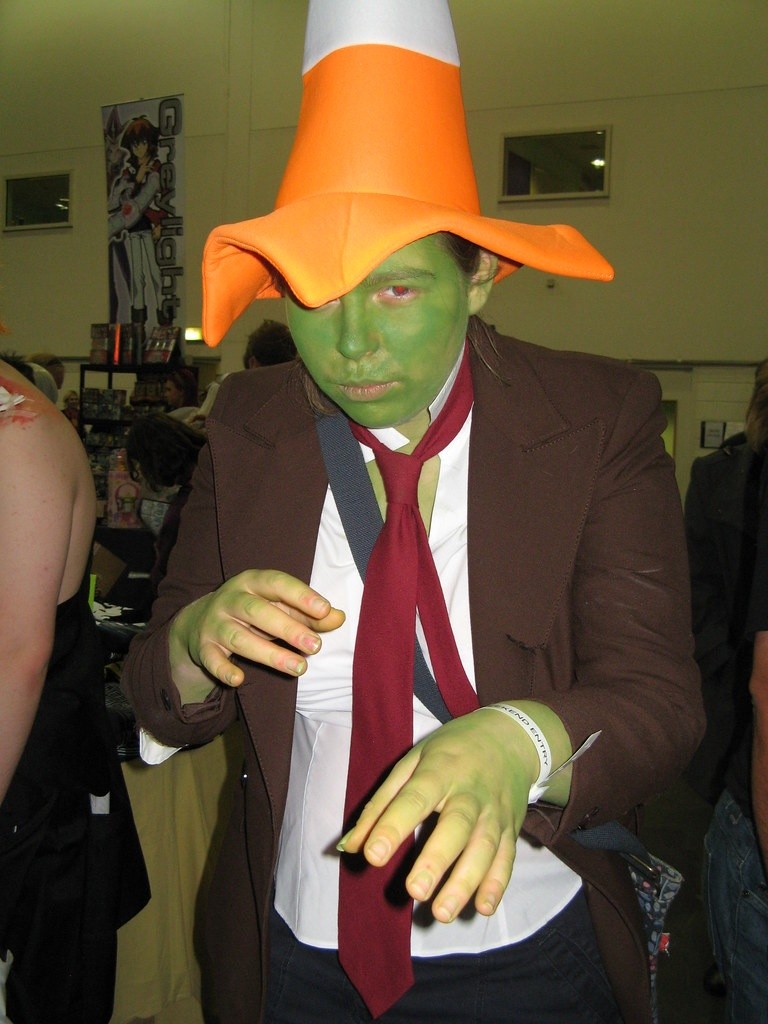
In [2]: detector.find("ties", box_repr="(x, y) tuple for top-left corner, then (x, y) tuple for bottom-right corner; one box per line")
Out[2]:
(316, 339), (475, 1019)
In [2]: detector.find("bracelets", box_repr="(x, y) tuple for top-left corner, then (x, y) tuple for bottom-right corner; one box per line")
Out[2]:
(475, 703), (554, 803)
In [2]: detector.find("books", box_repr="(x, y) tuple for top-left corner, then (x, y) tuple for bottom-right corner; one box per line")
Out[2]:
(79, 319), (186, 534)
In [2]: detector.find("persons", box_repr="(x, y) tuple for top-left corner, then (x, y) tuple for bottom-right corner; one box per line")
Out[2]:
(122, 318), (296, 605)
(677, 360), (768, 1023)
(0, 348), (82, 430)
(0, 359), (154, 1022)
(121, 194), (704, 1024)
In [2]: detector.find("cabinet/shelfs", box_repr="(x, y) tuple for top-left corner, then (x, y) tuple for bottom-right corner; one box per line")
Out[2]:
(78, 363), (199, 526)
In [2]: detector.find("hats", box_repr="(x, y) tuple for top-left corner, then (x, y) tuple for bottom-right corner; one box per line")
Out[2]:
(202, 0), (616, 349)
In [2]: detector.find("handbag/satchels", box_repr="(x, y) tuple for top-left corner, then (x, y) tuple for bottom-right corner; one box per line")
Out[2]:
(628, 853), (685, 1024)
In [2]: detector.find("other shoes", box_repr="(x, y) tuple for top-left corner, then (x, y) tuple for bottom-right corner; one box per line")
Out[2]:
(703, 964), (726, 998)
